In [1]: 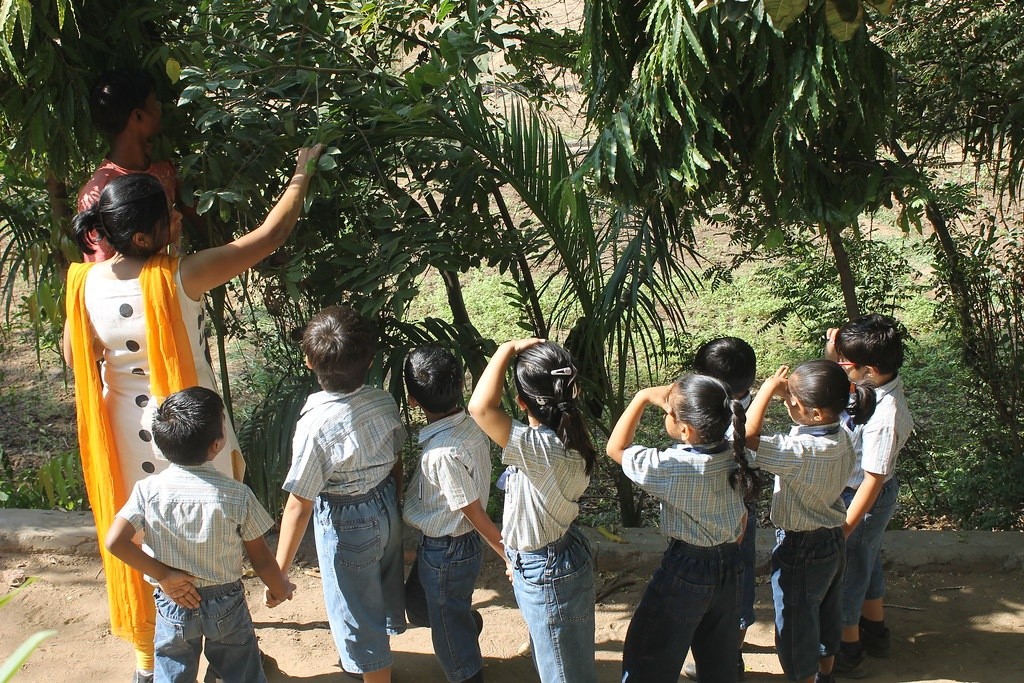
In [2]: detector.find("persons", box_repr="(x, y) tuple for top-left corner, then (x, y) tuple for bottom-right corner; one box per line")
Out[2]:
(403, 345), (511, 683)
(694, 337), (759, 643)
(104, 386), (297, 683)
(744, 360), (877, 683)
(262, 306), (409, 683)
(606, 375), (759, 683)
(824, 313), (914, 678)
(63, 65), (326, 683)
(468, 338), (597, 683)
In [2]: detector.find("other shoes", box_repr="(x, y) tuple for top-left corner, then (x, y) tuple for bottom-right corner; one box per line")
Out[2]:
(859, 624), (891, 659)
(686, 663), (698, 681)
(833, 650), (868, 679)
(472, 609), (484, 637)
(132, 670), (154, 683)
(815, 673), (835, 683)
(339, 658), (364, 680)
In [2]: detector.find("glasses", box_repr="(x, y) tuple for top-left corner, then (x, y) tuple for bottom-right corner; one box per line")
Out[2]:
(836, 355), (870, 367)
